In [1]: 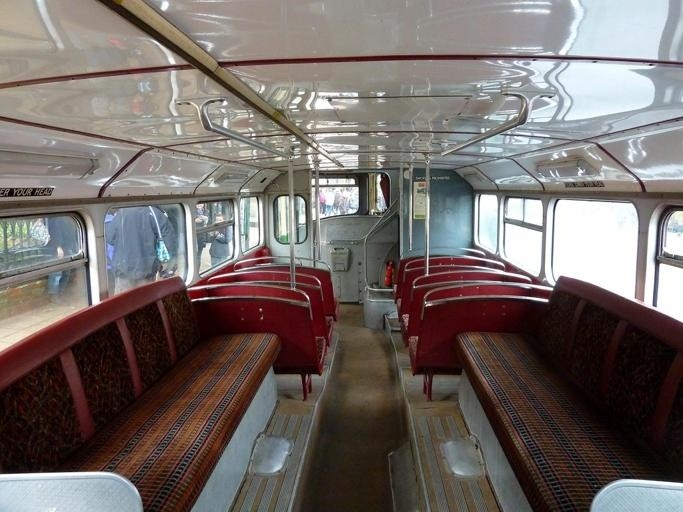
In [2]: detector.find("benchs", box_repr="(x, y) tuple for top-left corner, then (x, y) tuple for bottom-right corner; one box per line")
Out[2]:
(0, 275), (282, 511)
(184, 254), (340, 400)
(393, 245), (554, 402)
(456, 275), (683, 512)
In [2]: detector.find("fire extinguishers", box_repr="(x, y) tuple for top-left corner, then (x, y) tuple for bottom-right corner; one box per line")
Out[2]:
(383, 260), (395, 287)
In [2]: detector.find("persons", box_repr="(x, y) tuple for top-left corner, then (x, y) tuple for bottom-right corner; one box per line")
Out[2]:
(318, 187), (353, 217)
(30, 201), (232, 305)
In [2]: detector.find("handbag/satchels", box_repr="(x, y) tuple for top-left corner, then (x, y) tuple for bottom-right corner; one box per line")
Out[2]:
(156, 240), (170, 262)
